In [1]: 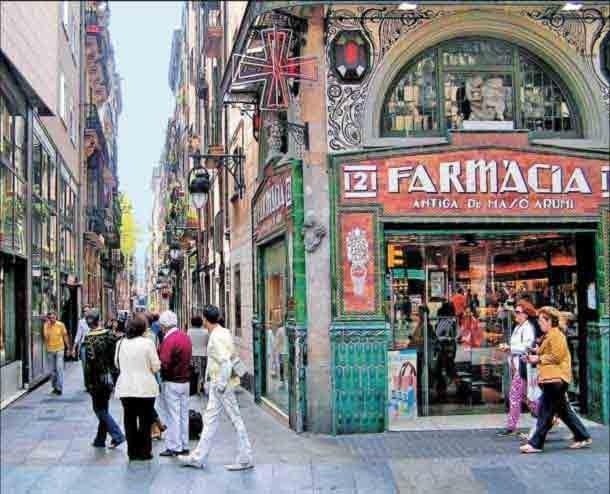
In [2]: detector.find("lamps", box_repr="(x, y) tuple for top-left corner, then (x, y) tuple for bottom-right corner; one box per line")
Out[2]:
(185, 150), (247, 210)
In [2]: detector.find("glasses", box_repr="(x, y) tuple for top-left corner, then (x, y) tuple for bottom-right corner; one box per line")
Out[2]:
(513, 310), (526, 314)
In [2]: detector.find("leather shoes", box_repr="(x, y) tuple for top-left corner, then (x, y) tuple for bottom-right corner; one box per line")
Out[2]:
(152, 423), (168, 441)
(159, 447), (190, 457)
(177, 454), (204, 468)
(223, 461), (255, 471)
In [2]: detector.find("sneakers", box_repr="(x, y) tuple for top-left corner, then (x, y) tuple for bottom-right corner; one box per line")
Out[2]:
(496, 427), (520, 438)
(129, 450), (154, 460)
(51, 387), (62, 395)
(568, 436), (593, 449)
(91, 434), (126, 448)
(519, 443), (545, 452)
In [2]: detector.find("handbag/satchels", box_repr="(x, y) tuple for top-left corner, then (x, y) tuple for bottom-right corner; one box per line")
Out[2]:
(112, 367), (120, 387)
(229, 353), (248, 380)
(526, 365), (544, 402)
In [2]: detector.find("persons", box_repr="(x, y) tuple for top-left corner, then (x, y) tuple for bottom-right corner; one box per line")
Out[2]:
(399, 286), (592, 454)
(41, 303), (255, 471)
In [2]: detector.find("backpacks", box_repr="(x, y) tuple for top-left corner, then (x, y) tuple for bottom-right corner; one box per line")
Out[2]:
(189, 408), (204, 440)
